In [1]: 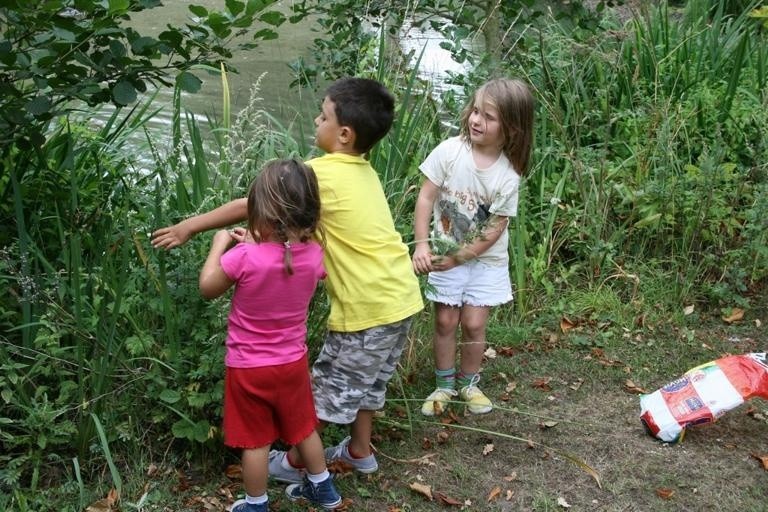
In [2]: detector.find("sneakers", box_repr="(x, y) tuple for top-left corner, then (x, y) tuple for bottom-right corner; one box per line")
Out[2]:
(420, 388), (454, 417)
(324, 439), (379, 474)
(285, 475), (342, 509)
(459, 386), (493, 415)
(231, 499), (270, 512)
(268, 450), (305, 484)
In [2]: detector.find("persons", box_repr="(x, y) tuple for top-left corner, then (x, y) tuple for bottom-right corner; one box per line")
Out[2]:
(148, 77), (427, 485)
(410, 75), (536, 418)
(196, 157), (343, 512)
